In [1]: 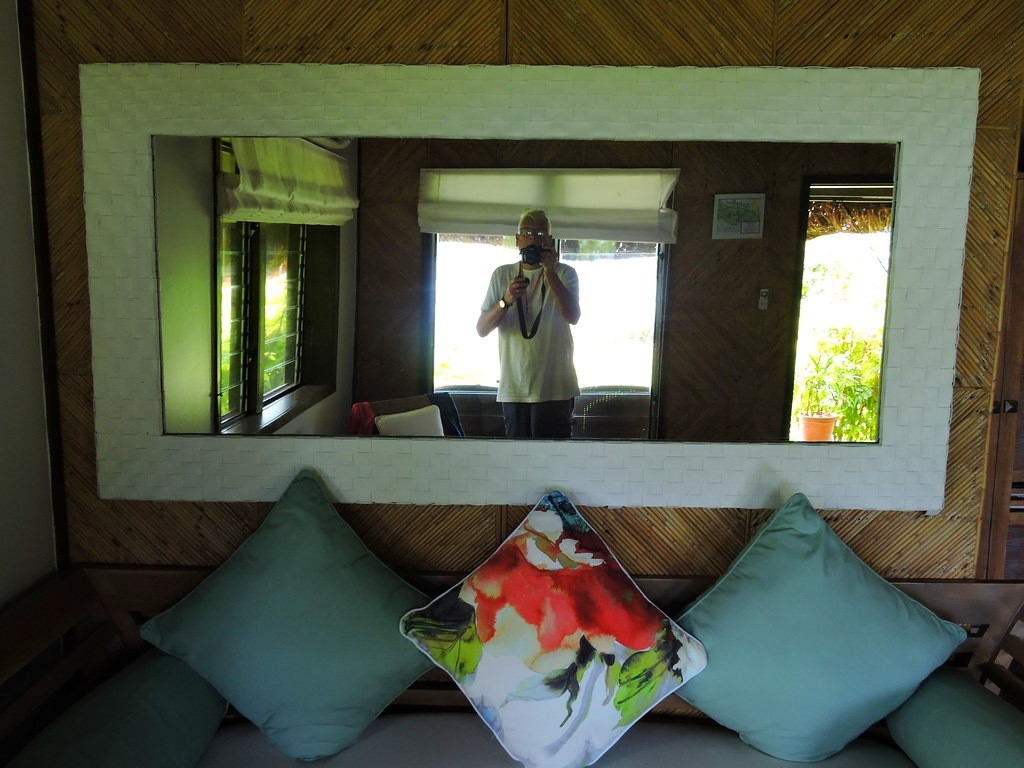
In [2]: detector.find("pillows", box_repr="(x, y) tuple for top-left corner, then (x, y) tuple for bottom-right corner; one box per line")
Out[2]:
(138, 475), (440, 761)
(669, 492), (967, 763)
(399, 491), (708, 768)
(374, 405), (444, 436)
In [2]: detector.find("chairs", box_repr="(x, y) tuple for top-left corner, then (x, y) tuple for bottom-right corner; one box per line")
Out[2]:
(352, 391), (465, 436)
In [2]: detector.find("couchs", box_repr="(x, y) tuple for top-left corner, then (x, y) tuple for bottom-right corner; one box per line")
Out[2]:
(0, 564), (1024, 768)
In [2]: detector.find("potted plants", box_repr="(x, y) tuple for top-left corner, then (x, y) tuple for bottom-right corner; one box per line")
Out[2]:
(791, 341), (849, 441)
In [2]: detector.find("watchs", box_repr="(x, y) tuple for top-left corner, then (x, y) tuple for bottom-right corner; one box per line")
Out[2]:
(499, 297), (511, 309)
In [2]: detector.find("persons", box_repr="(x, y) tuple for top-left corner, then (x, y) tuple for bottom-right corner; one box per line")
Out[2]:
(476, 209), (581, 439)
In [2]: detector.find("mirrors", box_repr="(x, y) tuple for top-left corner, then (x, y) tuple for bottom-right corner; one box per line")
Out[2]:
(77, 62), (982, 514)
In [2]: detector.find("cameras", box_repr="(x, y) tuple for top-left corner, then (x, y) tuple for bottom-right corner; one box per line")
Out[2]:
(521, 244), (546, 264)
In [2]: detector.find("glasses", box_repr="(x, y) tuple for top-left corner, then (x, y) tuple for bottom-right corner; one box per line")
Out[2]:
(517, 227), (550, 238)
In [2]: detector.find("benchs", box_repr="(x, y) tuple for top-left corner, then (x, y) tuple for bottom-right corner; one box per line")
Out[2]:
(433, 384), (649, 439)
